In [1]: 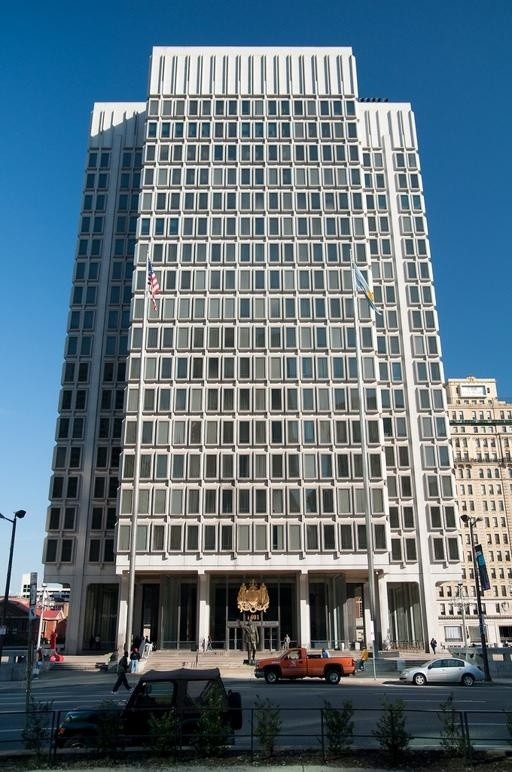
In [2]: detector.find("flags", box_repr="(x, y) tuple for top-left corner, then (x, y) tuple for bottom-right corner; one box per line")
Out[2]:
(351, 262), (382, 315)
(146, 258), (162, 313)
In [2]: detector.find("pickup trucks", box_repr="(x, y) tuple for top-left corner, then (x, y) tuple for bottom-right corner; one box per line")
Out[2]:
(254, 648), (355, 685)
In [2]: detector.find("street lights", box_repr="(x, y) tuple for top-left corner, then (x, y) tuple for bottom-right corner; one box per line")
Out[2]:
(460, 514), (492, 682)
(0, 509), (26, 668)
(35, 583), (49, 674)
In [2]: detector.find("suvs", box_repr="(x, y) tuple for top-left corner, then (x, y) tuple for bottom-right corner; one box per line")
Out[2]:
(54, 668), (242, 748)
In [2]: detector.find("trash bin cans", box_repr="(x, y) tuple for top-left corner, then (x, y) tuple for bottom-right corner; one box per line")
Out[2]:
(397, 660), (405, 672)
(33, 669), (38, 680)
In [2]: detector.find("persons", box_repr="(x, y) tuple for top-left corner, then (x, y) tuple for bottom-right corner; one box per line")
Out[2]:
(430, 638), (437, 654)
(130, 647), (142, 674)
(206, 633), (214, 651)
(357, 646), (368, 672)
(110, 651), (135, 694)
(138, 635), (150, 658)
(283, 632), (291, 653)
(320, 647), (329, 658)
(33, 648), (44, 680)
(238, 616), (260, 665)
(95, 634), (101, 651)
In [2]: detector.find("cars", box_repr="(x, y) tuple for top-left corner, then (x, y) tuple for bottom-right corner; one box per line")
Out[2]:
(399, 657), (485, 686)
